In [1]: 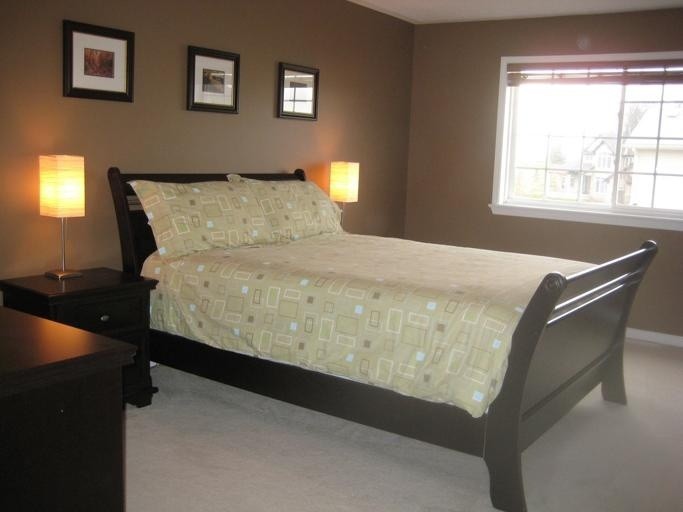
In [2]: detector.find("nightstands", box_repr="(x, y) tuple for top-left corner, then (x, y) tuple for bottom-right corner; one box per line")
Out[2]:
(1, 267), (160, 411)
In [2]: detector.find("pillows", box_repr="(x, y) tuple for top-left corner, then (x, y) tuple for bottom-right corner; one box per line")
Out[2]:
(126, 175), (344, 263)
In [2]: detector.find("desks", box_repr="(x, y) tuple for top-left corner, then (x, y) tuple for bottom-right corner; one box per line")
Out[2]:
(2, 308), (138, 512)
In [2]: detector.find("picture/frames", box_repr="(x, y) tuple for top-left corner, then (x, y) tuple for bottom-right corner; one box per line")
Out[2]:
(63, 19), (321, 120)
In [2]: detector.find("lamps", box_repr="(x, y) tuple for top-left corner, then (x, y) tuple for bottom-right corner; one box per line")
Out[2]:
(330, 160), (359, 225)
(38, 155), (86, 281)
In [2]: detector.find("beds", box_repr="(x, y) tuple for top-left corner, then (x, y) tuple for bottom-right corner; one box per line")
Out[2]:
(107, 166), (658, 511)
